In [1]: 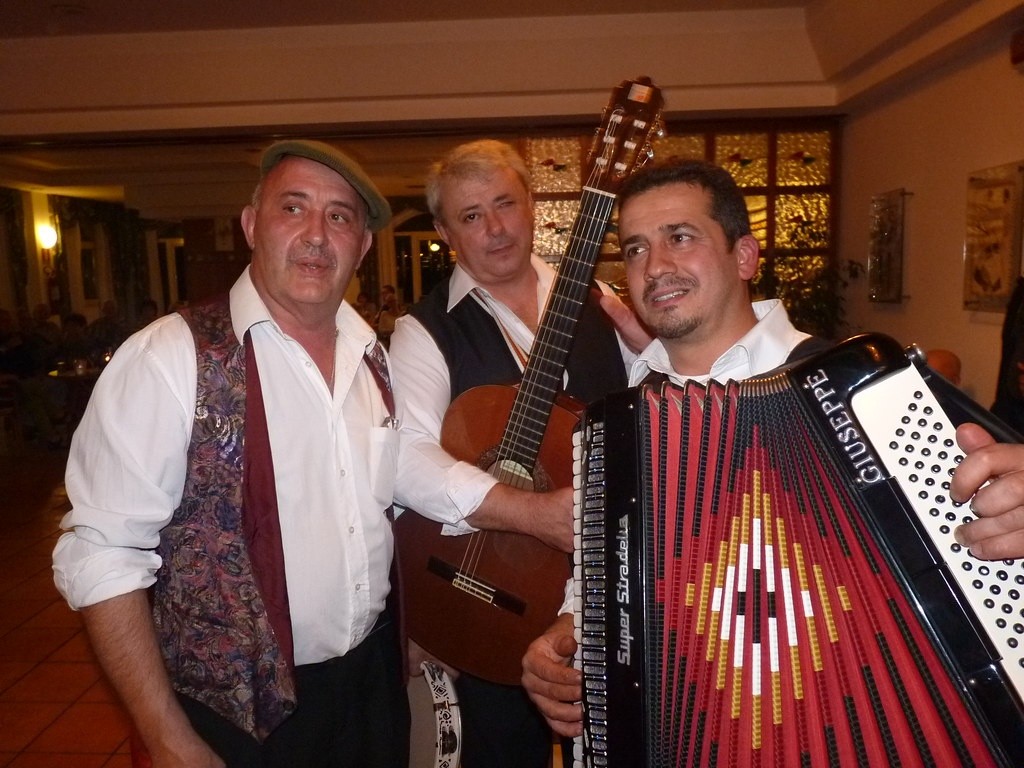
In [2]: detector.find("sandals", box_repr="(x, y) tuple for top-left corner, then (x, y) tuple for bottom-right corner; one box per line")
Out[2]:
(45, 436), (69, 451)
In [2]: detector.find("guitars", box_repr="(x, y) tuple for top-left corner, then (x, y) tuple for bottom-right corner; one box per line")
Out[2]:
(395, 75), (666, 689)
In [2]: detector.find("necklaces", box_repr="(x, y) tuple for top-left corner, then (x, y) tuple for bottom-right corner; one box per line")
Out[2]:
(329, 328), (338, 390)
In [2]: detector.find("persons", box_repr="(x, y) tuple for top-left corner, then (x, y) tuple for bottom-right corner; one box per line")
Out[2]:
(522, 158), (1024, 735)
(0, 300), (156, 448)
(351, 285), (406, 336)
(53, 138), (460, 768)
(389, 140), (653, 768)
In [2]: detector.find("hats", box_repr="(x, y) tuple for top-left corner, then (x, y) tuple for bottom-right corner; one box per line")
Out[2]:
(259, 138), (394, 234)
